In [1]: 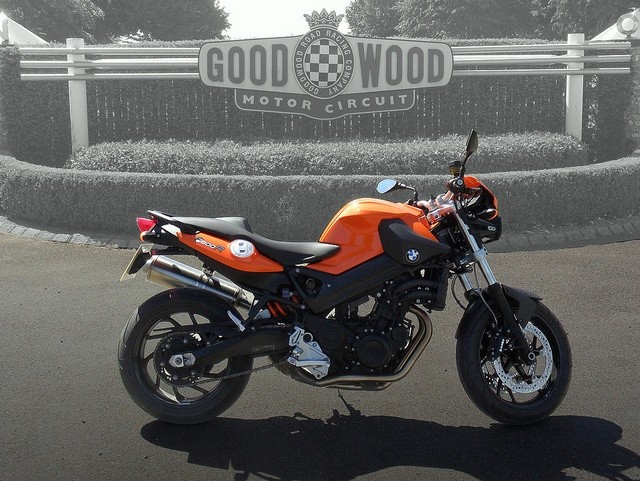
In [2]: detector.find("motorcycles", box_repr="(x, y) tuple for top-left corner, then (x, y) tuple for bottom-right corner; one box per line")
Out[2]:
(118, 129), (572, 424)
